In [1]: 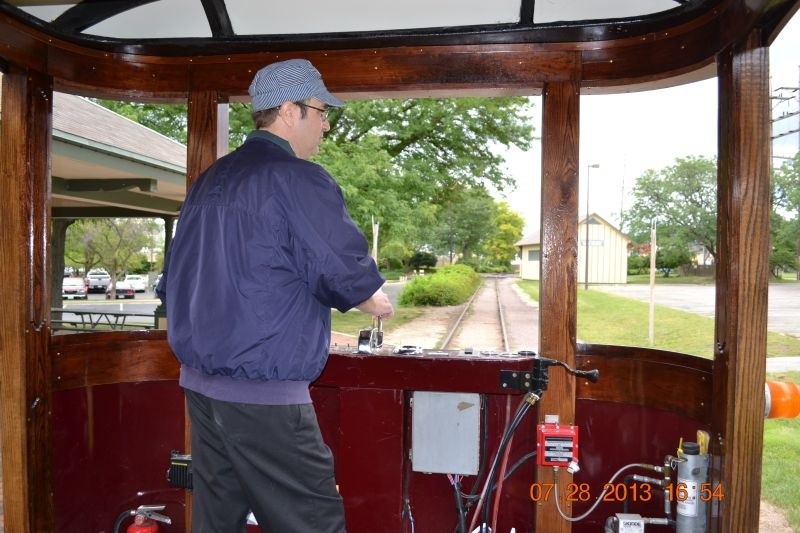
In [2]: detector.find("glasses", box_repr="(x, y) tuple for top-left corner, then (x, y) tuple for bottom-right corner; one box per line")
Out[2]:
(276, 102), (330, 120)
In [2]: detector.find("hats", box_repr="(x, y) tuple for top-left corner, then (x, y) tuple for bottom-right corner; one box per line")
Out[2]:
(250, 58), (346, 111)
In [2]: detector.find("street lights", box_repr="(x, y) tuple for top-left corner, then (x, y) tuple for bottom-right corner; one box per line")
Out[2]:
(585, 164), (600, 289)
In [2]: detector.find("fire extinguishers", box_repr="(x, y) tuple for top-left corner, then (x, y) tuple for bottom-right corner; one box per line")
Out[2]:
(114, 505), (171, 533)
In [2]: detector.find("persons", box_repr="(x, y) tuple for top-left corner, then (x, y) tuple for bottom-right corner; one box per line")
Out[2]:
(155, 59), (393, 533)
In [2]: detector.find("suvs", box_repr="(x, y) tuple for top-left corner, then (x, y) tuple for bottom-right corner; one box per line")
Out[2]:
(86, 270), (111, 290)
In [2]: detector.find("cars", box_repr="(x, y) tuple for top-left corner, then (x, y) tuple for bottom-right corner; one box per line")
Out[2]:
(124, 275), (145, 292)
(62, 278), (88, 300)
(107, 281), (134, 299)
